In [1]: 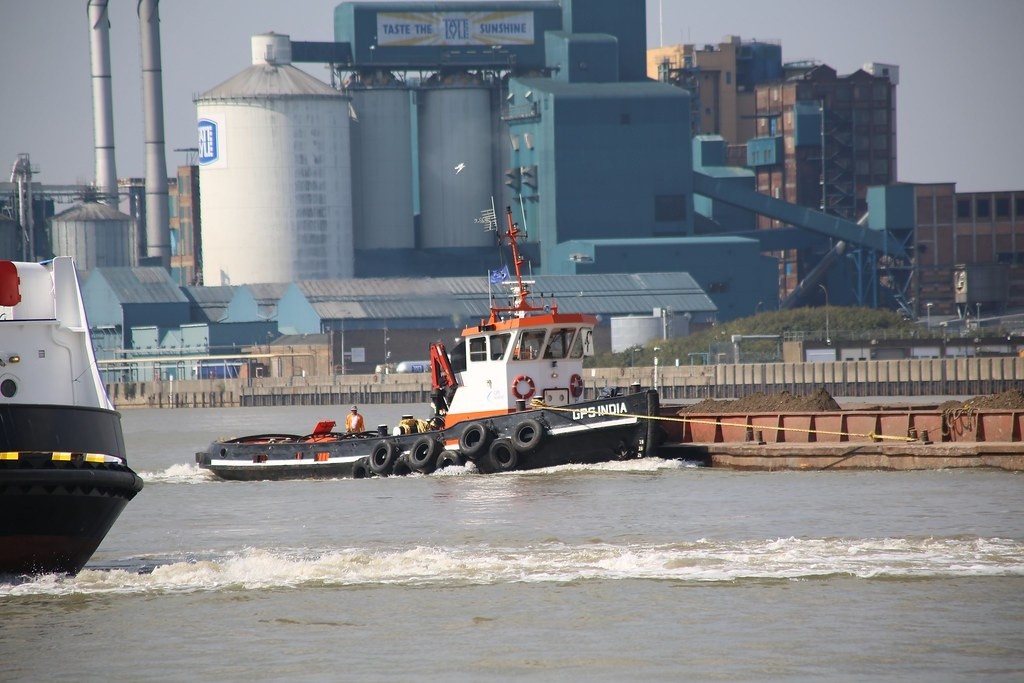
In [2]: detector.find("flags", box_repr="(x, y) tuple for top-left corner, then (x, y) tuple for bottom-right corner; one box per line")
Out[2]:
(491, 266), (510, 284)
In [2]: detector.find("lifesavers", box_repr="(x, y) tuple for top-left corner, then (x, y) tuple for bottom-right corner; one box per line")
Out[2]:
(570, 373), (583, 397)
(488, 437), (519, 471)
(367, 438), (400, 474)
(510, 418), (548, 457)
(351, 457), (374, 479)
(511, 375), (535, 399)
(409, 435), (442, 474)
(435, 449), (466, 472)
(458, 422), (494, 460)
(391, 454), (417, 476)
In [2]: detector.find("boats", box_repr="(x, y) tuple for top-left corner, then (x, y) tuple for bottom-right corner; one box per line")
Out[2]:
(0, 256), (144, 579)
(195, 203), (668, 482)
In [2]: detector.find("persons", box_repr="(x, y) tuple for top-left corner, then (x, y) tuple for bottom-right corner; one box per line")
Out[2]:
(346, 405), (365, 432)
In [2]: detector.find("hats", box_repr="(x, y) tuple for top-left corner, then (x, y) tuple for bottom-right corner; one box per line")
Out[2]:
(351, 406), (358, 410)
